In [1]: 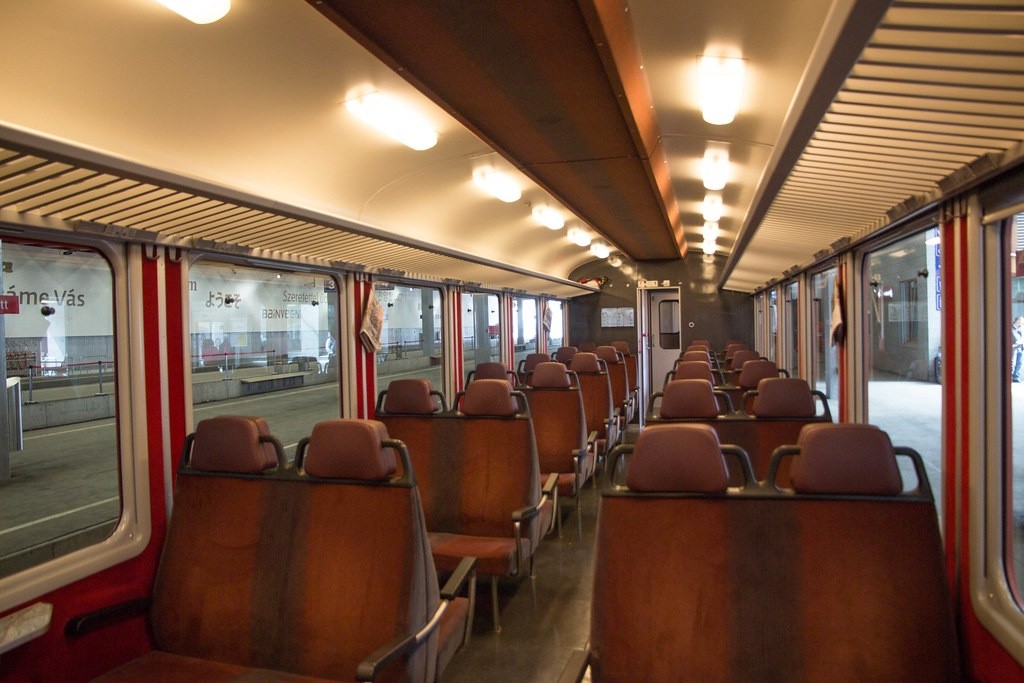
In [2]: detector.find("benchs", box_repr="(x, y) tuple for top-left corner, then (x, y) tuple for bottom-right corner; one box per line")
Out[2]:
(65, 341), (961, 683)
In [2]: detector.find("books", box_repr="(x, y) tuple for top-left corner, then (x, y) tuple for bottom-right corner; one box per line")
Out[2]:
(829, 280), (842, 348)
(543, 305), (552, 332)
(358, 288), (384, 354)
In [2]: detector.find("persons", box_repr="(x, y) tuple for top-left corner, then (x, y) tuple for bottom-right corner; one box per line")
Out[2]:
(490, 335), (499, 350)
(202, 339), (220, 366)
(1012, 314), (1024, 382)
(219, 333), (233, 367)
(325, 331), (338, 364)
